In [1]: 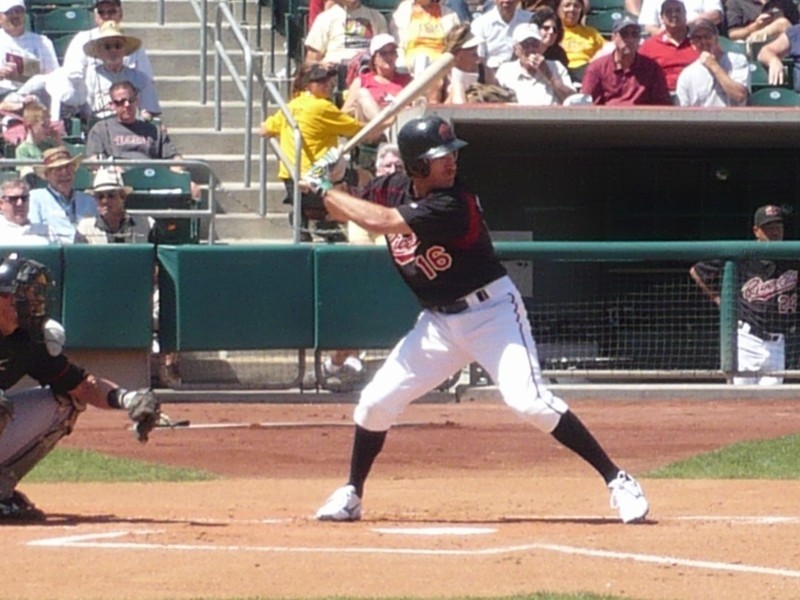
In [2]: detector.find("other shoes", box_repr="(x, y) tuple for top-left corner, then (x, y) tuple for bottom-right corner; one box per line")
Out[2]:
(0, 491), (45, 521)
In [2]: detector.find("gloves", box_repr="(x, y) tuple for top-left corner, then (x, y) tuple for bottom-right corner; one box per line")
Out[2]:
(302, 163), (332, 199)
(313, 146), (346, 185)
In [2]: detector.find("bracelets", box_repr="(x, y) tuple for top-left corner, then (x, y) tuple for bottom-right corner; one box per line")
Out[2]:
(315, 180), (333, 197)
(107, 386), (125, 410)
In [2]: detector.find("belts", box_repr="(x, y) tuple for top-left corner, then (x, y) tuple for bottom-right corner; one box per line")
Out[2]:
(737, 320), (779, 341)
(429, 290), (489, 314)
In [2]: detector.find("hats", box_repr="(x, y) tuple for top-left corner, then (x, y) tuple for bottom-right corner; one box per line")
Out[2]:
(83, 20), (143, 58)
(84, 165), (132, 194)
(33, 147), (82, 180)
(612, 15), (643, 32)
(0, 0), (25, 13)
(369, 33), (395, 56)
(754, 205), (783, 227)
(304, 63), (338, 81)
(688, 18), (716, 36)
(442, 25), (485, 49)
(88, 0), (121, 10)
(511, 23), (542, 44)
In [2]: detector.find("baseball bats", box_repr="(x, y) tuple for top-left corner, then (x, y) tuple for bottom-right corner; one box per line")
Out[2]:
(298, 52), (455, 193)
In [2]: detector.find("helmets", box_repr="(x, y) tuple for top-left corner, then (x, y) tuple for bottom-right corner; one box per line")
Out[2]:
(398, 117), (468, 178)
(0, 253), (45, 295)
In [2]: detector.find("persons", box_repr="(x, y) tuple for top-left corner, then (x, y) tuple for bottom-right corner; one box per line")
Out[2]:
(74, 165), (168, 245)
(87, 80), (203, 202)
(296, 116), (651, 524)
(256, 62), (382, 242)
(1, 254), (163, 524)
(675, 18), (751, 108)
(686, 204), (800, 385)
(256, 0), (800, 242)
(495, 22), (577, 105)
(580, 16), (672, 105)
(0, 0), (203, 245)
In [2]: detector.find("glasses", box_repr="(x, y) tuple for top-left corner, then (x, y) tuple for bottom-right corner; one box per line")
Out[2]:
(691, 34), (713, 40)
(378, 50), (396, 56)
(113, 98), (136, 106)
(99, 43), (125, 50)
(95, 193), (114, 199)
(2, 195), (28, 203)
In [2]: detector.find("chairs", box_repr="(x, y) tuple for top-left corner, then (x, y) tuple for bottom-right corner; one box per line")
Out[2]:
(585, 0), (800, 108)
(20, 0), (200, 246)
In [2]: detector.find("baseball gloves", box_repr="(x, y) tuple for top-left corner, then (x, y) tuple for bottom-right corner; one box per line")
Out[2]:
(129, 389), (161, 442)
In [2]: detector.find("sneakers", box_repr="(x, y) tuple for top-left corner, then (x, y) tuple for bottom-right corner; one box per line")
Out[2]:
(317, 485), (361, 521)
(607, 470), (649, 523)
